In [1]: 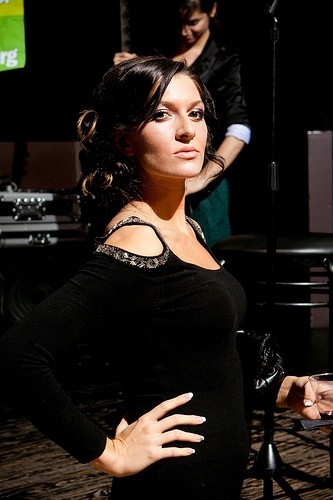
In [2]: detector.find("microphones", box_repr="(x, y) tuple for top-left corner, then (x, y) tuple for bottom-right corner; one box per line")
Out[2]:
(263, 0), (280, 20)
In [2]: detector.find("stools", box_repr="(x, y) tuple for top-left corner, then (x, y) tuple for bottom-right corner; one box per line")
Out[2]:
(216, 231), (333, 500)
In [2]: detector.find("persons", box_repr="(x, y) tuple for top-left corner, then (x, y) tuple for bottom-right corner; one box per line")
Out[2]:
(110, 0), (231, 243)
(21, 58), (332, 500)
(180, 0), (252, 248)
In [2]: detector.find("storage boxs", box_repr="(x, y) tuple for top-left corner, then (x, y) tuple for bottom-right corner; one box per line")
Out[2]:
(0, 140), (86, 192)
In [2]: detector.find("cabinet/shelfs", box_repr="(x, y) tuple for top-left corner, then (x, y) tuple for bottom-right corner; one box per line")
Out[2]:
(303, 128), (333, 331)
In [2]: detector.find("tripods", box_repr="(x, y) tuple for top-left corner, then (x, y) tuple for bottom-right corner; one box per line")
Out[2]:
(245, 24), (332, 500)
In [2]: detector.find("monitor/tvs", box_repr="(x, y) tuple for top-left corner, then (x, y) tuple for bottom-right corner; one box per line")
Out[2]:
(0, 0), (27, 71)
